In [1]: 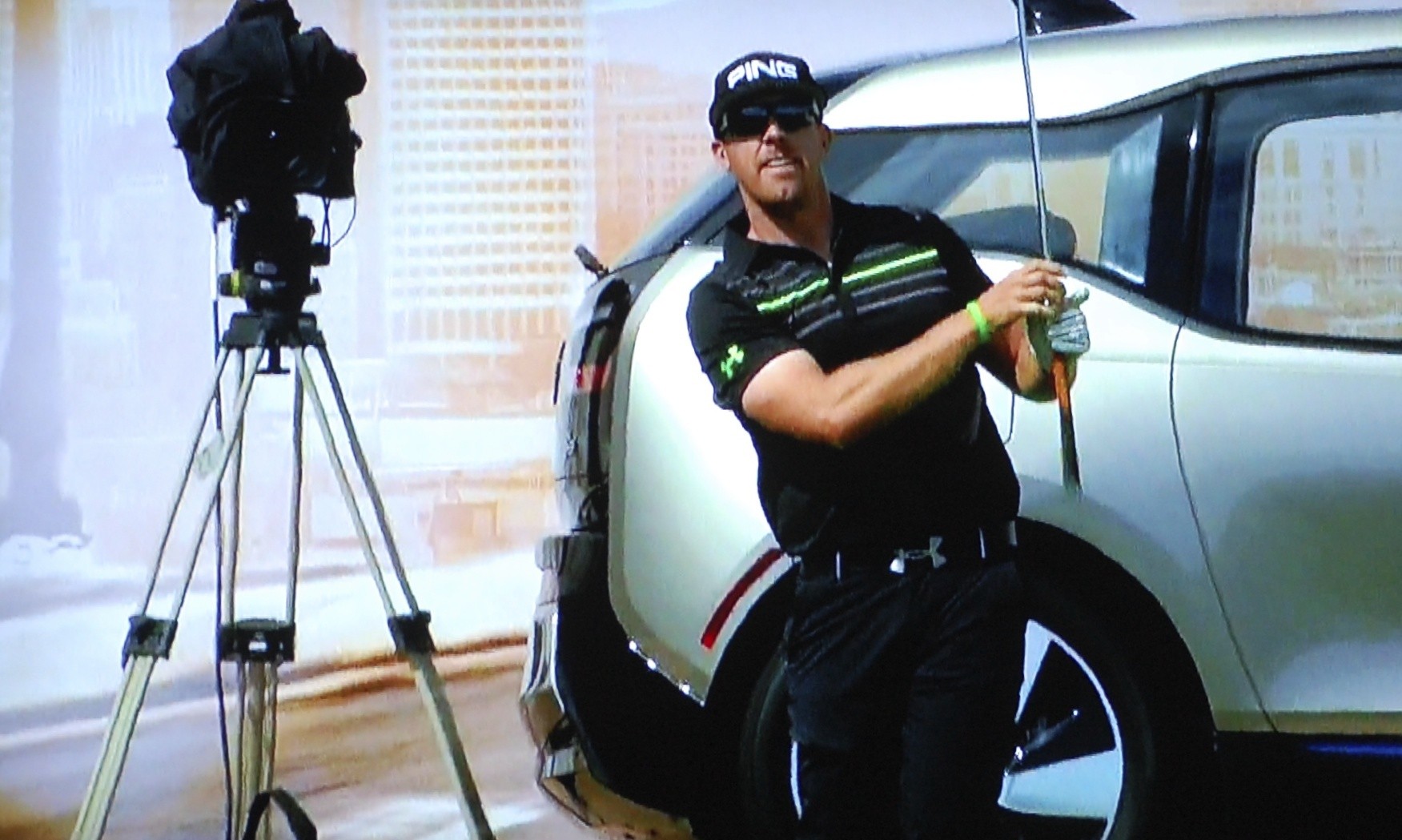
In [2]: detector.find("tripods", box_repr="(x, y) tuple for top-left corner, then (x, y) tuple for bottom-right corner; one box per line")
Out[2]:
(66, 218), (496, 840)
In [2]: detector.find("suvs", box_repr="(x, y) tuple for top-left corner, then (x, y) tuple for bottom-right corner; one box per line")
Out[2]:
(515, 12), (1402, 840)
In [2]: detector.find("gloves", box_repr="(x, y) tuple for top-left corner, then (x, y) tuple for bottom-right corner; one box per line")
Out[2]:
(1026, 288), (1090, 375)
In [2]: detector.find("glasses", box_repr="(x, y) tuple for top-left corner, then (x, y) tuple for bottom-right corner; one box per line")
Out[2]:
(719, 102), (821, 145)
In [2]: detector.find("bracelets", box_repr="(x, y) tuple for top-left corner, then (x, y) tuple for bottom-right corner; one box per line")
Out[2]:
(966, 301), (992, 343)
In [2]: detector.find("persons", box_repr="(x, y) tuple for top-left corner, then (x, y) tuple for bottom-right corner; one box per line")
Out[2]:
(686, 52), (1091, 840)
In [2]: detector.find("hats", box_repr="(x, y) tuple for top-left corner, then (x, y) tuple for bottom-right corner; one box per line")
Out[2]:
(709, 53), (828, 138)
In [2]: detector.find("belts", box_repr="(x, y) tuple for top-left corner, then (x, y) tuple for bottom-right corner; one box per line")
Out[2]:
(802, 520), (1016, 580)
